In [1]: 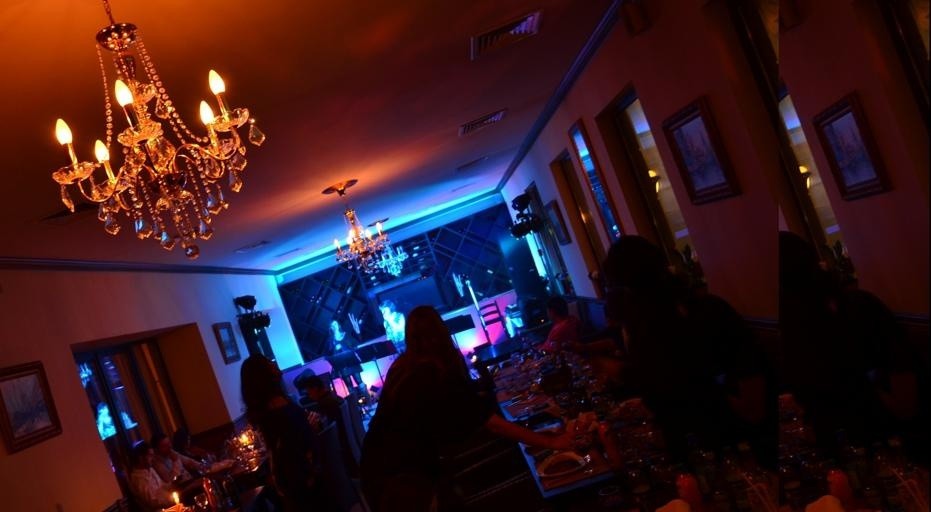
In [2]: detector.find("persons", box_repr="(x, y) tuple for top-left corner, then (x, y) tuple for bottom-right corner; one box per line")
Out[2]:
(600, 237), (781, 470)
(325, 320), (363, 395)
(538, 298), (577, 356)
(773, 231), (931, 450)
(360, 306), (577, 509)
(103, 426), (218, 512)
(379, 302), (407, 352)
(241, 352), (335, 511)
(298, 373), (361, 502)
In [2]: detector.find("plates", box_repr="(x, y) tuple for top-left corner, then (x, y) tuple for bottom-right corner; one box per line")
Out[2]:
(536, 454), (586, 477)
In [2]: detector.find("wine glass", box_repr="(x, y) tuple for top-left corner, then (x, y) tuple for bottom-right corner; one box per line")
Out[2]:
(194, 493), (209, 512)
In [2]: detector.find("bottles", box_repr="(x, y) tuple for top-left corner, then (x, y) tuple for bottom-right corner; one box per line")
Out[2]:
(775, 392), (822, 464)
(202, 474), (242, 511)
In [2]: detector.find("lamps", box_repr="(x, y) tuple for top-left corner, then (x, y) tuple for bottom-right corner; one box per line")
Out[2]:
(322, 179), (390, 272)
(51, 0), (266, 261)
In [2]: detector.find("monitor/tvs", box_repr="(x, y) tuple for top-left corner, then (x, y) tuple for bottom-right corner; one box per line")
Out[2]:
(375, 274), (448, 317)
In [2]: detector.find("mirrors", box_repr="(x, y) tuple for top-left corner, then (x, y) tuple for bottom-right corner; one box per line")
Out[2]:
(567, 118), (625, 246)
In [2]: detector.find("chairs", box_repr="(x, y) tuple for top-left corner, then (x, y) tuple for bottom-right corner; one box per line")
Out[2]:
(312, 392), (366, 512)
(477, 300), (510, 344)
(439, 437), (532, 512)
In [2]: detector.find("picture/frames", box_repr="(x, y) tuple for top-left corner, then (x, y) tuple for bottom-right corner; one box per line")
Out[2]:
(0, 360), (63, 454)
(213, 322), (241, 365)
(660, 96), (741, 205)
(810, 92), (890, 201)
(545, 200), (571, 245)
